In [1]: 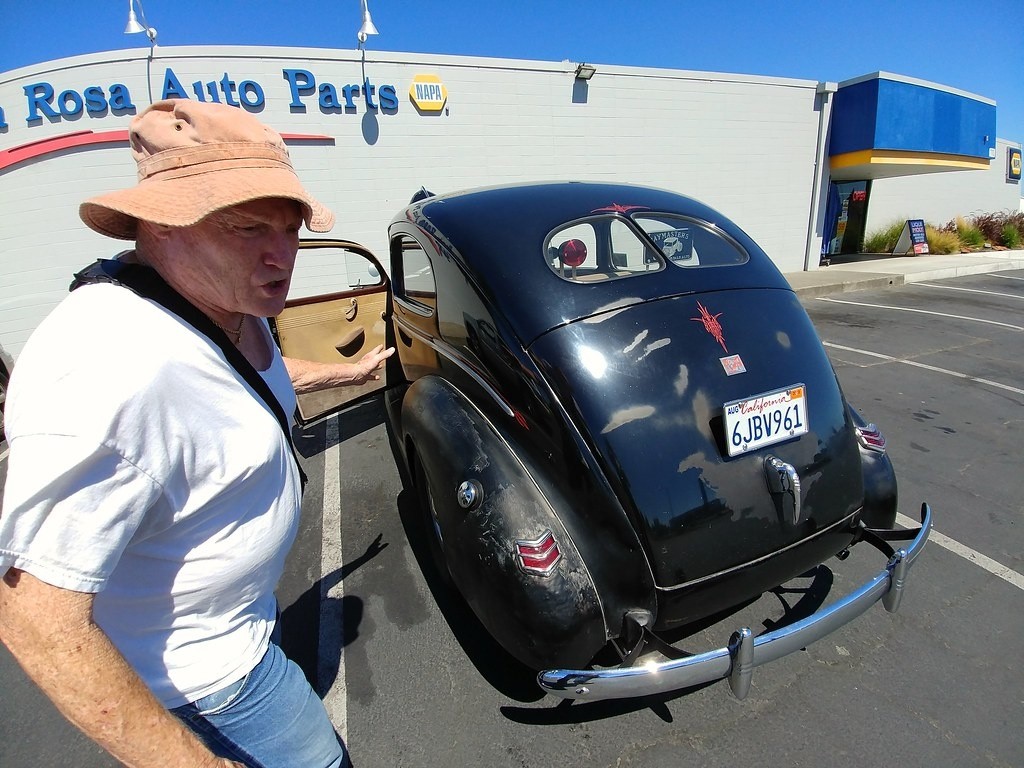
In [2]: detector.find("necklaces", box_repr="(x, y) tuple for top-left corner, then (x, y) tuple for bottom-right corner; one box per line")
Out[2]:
(204, 312), (247, 344)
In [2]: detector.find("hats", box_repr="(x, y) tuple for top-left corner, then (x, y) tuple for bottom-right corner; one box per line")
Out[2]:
(78, 98), (336, 240)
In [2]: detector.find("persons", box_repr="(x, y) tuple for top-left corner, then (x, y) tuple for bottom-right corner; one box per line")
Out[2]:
(0, 99), (395, 768)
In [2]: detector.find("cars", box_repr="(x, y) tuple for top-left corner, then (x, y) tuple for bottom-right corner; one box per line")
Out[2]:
(268, 182), (934, 700)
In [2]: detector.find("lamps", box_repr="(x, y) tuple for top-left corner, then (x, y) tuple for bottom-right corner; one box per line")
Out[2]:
(124, 0), (157, 39)
(574, 63), (596, 80)
(357, 0), (380, 44)
